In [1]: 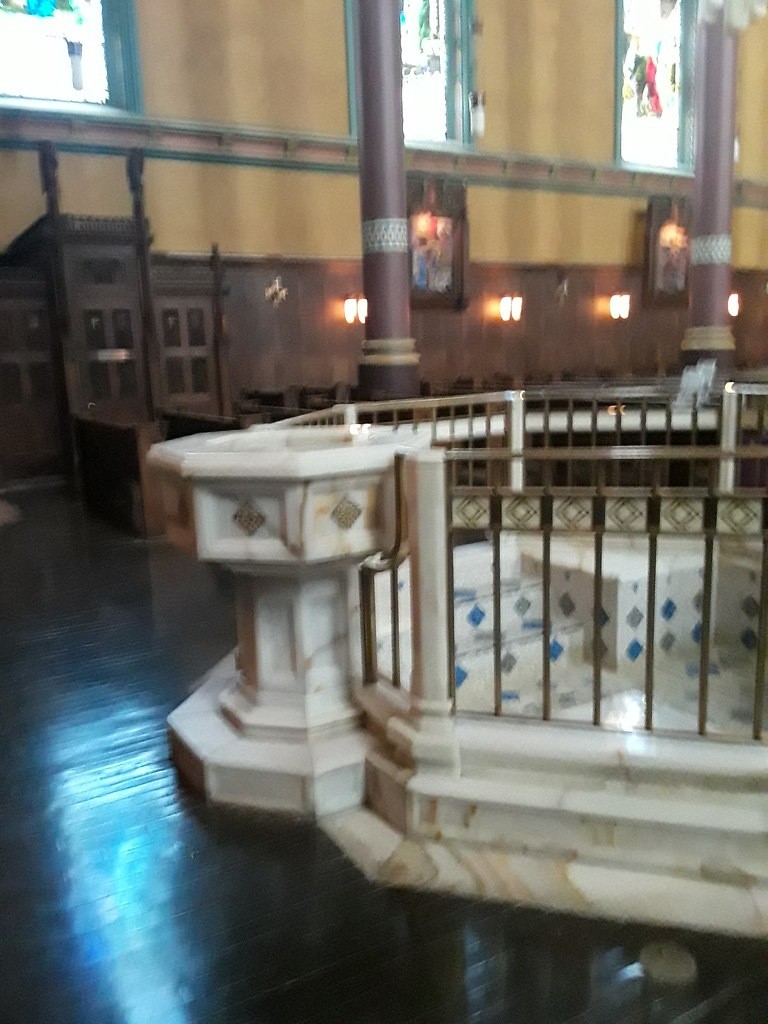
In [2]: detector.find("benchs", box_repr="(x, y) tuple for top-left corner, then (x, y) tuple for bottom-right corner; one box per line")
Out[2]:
(75, 365), (768, 538)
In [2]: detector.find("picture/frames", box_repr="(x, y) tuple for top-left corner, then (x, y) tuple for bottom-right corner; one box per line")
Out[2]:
(642, 194), (694, 309)
(407, 170), (474, 310)
